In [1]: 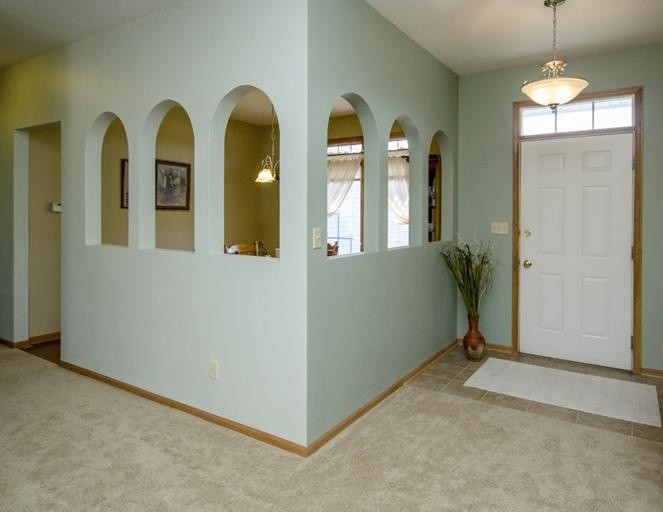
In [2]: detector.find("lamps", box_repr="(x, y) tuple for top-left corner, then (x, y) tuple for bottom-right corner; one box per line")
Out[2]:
(255, 105), (280, 183)
(520, 0), (590, 113)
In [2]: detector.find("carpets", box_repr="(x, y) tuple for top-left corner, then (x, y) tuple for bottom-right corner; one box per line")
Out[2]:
(463, 356), (661, 428)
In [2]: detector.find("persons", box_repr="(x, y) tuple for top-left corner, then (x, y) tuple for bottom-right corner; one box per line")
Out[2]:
(160, 166), (186, 202)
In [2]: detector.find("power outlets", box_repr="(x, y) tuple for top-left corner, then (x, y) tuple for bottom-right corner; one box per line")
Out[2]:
(491, 222), (508, 234)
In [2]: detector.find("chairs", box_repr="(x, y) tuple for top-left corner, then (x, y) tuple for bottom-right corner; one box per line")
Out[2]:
(224, 240), (258, 256)
(326, 240), (339, 256)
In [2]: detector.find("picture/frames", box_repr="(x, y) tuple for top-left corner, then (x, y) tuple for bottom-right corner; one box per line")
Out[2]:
(120, 159), (128, 209)
(155, 159), (191, 210)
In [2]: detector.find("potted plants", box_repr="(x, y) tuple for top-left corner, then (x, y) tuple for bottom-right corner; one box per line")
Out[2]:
(440, 232), (499, 362)
